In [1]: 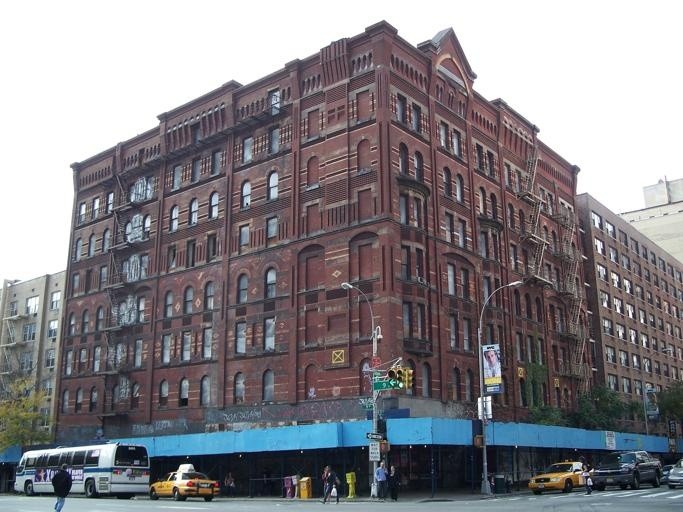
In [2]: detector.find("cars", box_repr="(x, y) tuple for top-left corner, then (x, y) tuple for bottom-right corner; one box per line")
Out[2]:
(666, 458), (682, 488)
(527, 458), (597, 494)
(149, 463), (221, 501)
(659, 464), (674, 483)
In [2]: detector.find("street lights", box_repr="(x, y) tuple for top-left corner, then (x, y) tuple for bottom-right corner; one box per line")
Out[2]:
(476, 280), (526, 494)
(340, 281), (382, 499)
(642, 347), (673, 452)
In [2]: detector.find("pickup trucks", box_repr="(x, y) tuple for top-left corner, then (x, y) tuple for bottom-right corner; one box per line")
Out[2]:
(590, 449), (661, 490)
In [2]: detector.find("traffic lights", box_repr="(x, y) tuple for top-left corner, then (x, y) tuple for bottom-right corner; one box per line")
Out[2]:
(407, 370), (415, 388)
(388, 370), (396, 387)
(396, 368), (404, 389)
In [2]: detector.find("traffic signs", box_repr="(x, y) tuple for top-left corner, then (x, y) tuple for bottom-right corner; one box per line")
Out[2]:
(373, 371), (382, 376)
(361, 405), (374, 411)
(366, 433), (383, 440)
(359, 398), (374, 405)
(373, 380), (400, 391)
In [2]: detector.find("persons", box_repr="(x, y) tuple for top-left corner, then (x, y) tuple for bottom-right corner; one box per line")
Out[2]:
(224, 472), (234, 498)
(319, 465), (340, 505)
(259, 466), (274, 498)
(321, 466), (330, 503)
(386, 465), (401, 503)
(577, 456), (592, 496)
(48, 463), (72, 511)
(483, 352), (500, 382)
(373, 461), (388, 503)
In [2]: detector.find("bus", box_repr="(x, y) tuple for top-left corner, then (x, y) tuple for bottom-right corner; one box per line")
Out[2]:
(12, 443), (150, 499)
